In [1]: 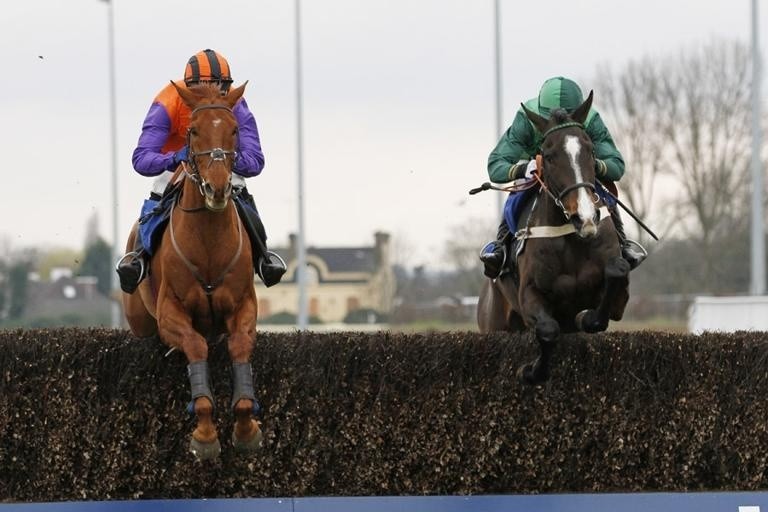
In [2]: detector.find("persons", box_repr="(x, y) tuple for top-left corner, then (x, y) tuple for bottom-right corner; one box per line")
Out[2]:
(116, 49), (286, 295)
(481, 77), (648, 278)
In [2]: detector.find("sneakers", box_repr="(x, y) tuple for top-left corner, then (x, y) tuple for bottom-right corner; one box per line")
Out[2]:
(253, 257), (287, 289)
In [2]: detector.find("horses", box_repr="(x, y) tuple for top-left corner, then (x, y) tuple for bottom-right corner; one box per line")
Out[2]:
(121, 79), (263, 461)
(476, 88), (630, 386)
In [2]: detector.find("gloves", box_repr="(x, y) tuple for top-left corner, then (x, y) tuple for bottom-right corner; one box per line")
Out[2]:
(524, 158), (538, 179)
(175, 145), (188, 163)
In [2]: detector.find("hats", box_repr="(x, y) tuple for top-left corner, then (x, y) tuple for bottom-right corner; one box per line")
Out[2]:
(535, 76), (584, 119)
(182, 48), (235, 85)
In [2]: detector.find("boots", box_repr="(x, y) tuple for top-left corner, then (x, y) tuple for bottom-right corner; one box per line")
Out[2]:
(478, 217), (515, 279)
(115, 223), (149, 295)
(606, 204), (645, 270)
